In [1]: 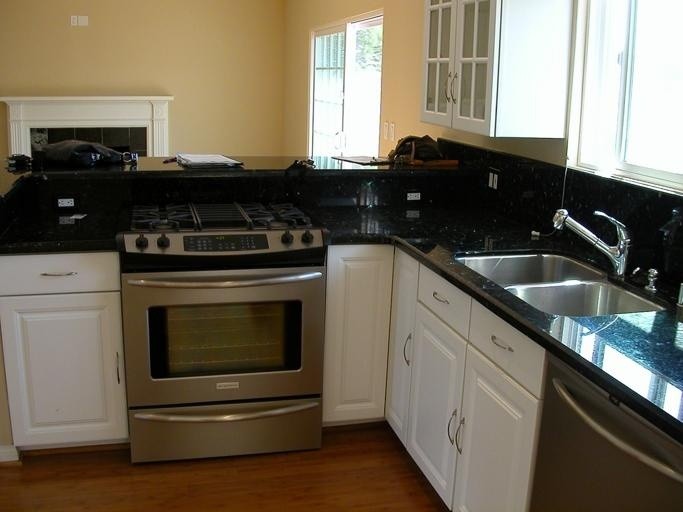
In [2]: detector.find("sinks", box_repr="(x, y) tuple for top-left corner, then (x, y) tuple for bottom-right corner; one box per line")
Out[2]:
(456, 247), (607, 288)
(500, 281), (668, 319)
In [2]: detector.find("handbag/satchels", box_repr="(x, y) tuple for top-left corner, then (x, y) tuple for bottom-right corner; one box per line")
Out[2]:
(39, 138), (141, 171)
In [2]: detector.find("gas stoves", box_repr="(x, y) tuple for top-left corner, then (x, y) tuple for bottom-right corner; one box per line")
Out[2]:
(115, 198), (327, 270)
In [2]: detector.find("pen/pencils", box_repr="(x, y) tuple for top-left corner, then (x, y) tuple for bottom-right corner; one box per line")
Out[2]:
(162, 158), (176, 163)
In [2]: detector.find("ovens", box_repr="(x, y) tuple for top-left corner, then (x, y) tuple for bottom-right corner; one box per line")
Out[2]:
(119, 264), (326, 465)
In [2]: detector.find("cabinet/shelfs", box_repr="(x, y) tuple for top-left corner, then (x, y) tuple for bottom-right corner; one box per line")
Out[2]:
(1, 251), (132, 456)
(385, 246), (419, 449)
(406, 258), (547, 511)
(418, 0), (572, 139)
(323, 243), (397, 426)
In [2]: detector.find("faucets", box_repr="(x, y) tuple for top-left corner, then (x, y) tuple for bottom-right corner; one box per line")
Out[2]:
(551, 209), (617, 259)
